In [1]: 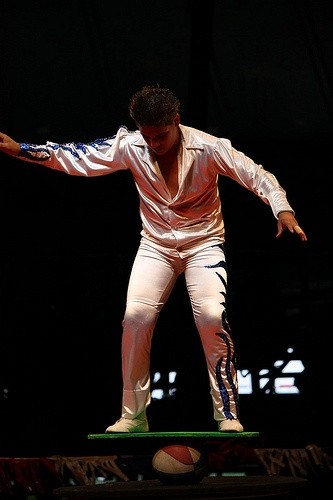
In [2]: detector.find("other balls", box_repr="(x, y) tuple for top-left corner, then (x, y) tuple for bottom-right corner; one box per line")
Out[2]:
(152, 443), (209, 484)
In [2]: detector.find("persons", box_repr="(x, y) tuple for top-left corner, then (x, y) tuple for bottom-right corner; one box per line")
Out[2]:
(0, 86), (308, 432)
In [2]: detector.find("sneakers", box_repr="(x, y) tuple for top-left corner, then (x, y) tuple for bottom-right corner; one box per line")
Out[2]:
(105, 419), (149, 432)
(219, 419), (244, 432)
